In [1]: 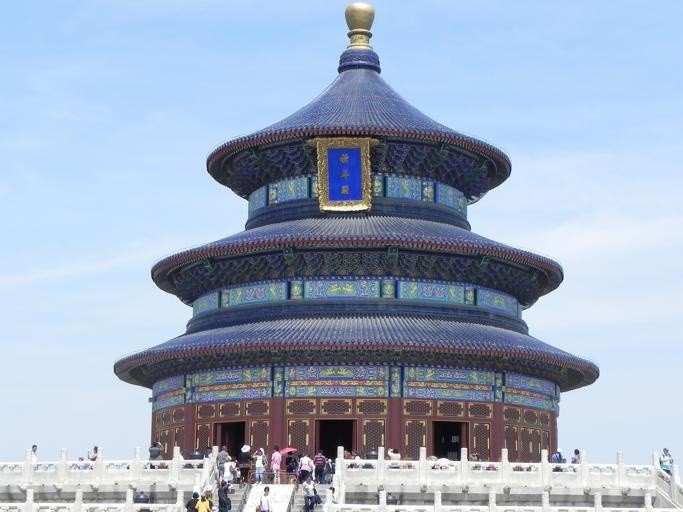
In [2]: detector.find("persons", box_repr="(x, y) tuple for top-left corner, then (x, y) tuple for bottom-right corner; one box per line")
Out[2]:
(32, 445), (38, 470)
(474, 454), (479, 461)
(149, 440), (164, 468)
(388, 448), (402, 468)
(571, 449), (580, 471)
(660, 447), (674, 474)
(186, 445), (364, 512)
(88, 446), (98, 468)
(552, 448), (562, 472)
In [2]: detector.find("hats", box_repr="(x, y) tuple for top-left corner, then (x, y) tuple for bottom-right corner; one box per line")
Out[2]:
(241, 445), (250, 453)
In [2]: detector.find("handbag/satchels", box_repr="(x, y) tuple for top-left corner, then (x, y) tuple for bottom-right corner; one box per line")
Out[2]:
(315, 495), (322, 506)
(256, 506), (260, 512)
(229, 465), (236, 476)
(267, 470), (273, 481)
(288, 457), (295, 469)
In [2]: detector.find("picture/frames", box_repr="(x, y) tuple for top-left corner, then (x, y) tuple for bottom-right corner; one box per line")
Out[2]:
(316, 137), (371, 212)
